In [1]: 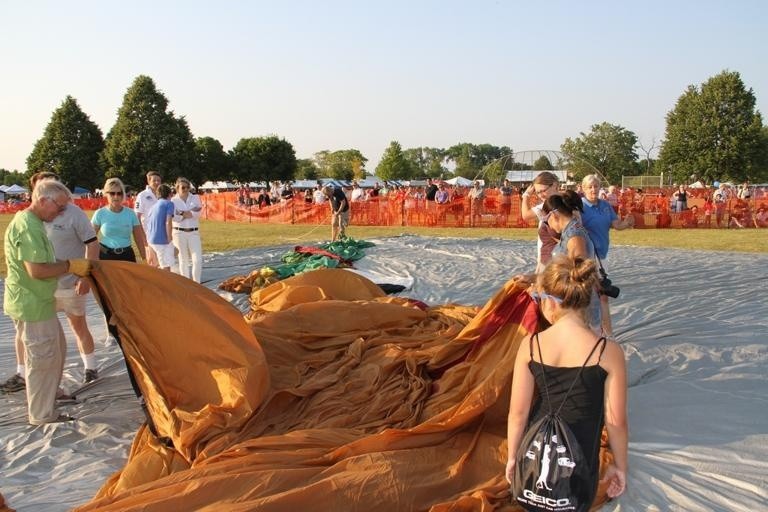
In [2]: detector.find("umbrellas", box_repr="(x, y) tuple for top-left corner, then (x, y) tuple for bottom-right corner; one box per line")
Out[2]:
(0, 183), (29, 205)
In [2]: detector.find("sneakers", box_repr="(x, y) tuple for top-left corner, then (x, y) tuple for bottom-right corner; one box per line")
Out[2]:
(0, 374), (26, 392)
(83, 368), (97, 383)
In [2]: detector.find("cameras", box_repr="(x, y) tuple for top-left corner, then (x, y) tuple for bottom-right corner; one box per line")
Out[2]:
(597, 279), (620, 298)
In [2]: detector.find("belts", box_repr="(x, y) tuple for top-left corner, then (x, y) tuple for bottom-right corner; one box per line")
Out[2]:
(174, 227), (198, 232)
(109, 248), (128, 254)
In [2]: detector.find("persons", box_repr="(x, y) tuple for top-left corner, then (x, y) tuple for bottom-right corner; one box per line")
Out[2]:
(0, 170), (100, 395)
(236, 181), (327, 222)
(513, 189), (606, 339)
(320, 183), (351, 243)
(171, 175), (204, 287)
(519, 170), (582, 278)
(600, 185), (647, 226)
(653, 179), (767, 229)
(147, 183), (179, 275)
(133, 171), (163, 268)
(90, 177), (149, 270)
(341, 178), (530, 226)
(2, 177), (102, 427)
(576, 171), (635, 338)
(504, 253), (630, 511)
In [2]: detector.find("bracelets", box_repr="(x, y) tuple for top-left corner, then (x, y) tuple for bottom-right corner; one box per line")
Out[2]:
(141, 256), (147, 260)
(179, 211), (184, 215)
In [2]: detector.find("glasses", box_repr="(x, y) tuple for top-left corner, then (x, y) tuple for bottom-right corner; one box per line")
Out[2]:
(543, 209), (558, 225)
(532, 290), (561, 305)
(107, 192), (122, 196)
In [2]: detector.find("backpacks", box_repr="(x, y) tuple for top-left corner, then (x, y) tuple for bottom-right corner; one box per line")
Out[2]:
(511, 333), (604, 512)
